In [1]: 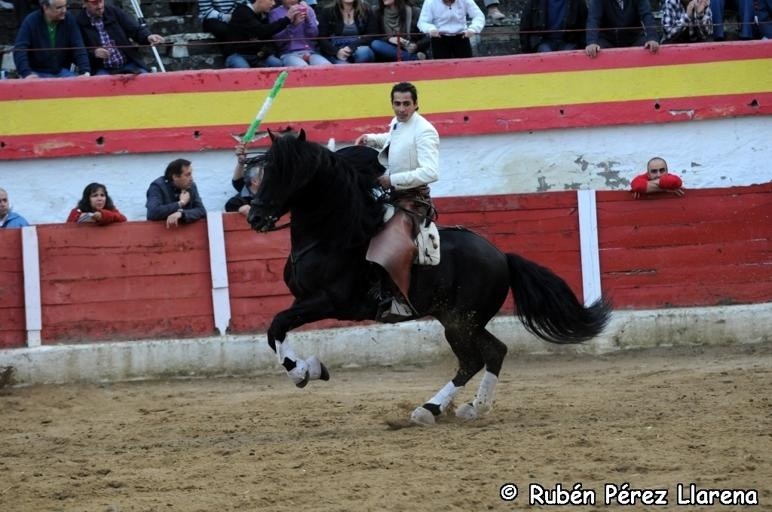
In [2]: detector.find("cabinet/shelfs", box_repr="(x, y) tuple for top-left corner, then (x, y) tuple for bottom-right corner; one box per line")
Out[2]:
(304, 45), (311, 64)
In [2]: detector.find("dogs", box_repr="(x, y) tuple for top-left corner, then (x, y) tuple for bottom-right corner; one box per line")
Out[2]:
(248, 127), (617, 428)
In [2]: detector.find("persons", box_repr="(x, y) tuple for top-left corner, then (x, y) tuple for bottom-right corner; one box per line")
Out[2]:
(144, 158), (207, 230)
(13, 0), (772, 81)
(65, 182), (127, 225)
(231, 144), (248, 193)
(224, 164), (264, 217)
(629, 156), (686, 200)
(0, 187), (30, 228)
(352, 82), (440, 322)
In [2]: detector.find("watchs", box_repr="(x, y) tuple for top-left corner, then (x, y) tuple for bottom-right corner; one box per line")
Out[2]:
(177, 208), (183, 212)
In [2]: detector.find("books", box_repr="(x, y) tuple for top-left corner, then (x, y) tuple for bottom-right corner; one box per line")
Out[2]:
(77, 212), (96, 224)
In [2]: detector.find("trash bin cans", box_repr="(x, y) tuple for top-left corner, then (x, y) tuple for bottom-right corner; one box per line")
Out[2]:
(177, 208), (183, 212)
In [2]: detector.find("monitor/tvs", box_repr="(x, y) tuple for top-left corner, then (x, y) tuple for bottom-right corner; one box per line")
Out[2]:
(77, 212), (96, 224)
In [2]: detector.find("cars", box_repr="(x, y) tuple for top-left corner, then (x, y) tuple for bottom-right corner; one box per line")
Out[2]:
(299, 5), (307, 17)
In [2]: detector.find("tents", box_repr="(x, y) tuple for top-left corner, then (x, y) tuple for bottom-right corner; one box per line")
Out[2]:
(243, 164), (253, 187)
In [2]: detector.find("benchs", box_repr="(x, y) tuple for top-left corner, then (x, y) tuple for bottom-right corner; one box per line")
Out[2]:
(1, 0), (531, 79)
